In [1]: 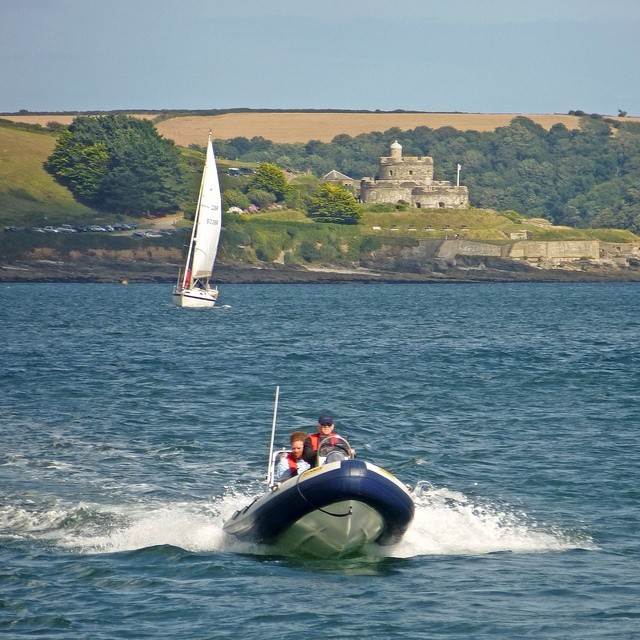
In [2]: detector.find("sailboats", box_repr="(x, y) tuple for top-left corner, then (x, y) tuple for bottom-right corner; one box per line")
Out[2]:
(173, 130), (224, 309)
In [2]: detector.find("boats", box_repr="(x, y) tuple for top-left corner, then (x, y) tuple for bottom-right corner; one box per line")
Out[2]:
(222, 383), (416, 558)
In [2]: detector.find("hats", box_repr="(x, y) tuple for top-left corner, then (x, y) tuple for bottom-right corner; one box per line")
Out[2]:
(319, 415), (333, 424)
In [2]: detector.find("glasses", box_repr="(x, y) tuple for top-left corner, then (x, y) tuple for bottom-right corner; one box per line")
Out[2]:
(323, 423), (332, 426)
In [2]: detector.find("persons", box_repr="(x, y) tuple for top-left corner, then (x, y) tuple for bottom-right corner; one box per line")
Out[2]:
(303, 415), (356, 467)
(277, 432), (311, 482)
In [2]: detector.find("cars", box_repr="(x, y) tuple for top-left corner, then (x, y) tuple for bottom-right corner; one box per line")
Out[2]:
(2, 221), (138, 237)
(130, 226), (173, 239)
(172, 226), (192, 238)
